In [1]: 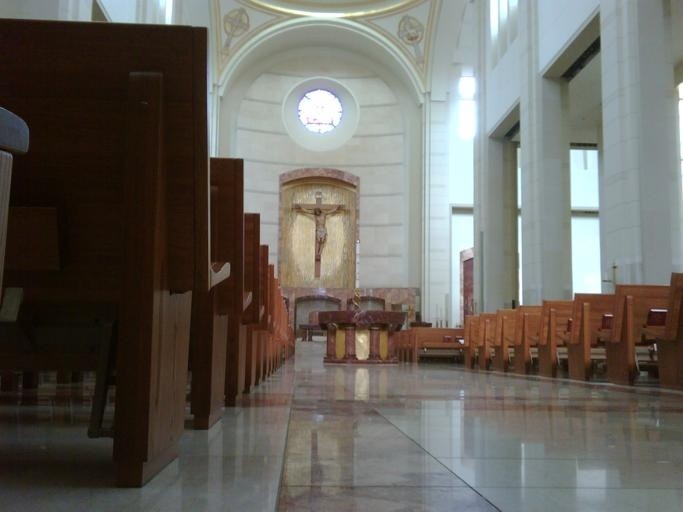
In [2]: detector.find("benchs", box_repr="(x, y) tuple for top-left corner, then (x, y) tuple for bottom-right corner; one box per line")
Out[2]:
(395, 269), (681, 389)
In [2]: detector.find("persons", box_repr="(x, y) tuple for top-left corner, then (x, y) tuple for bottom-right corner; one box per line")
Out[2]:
(294, 203), (341, 262)
